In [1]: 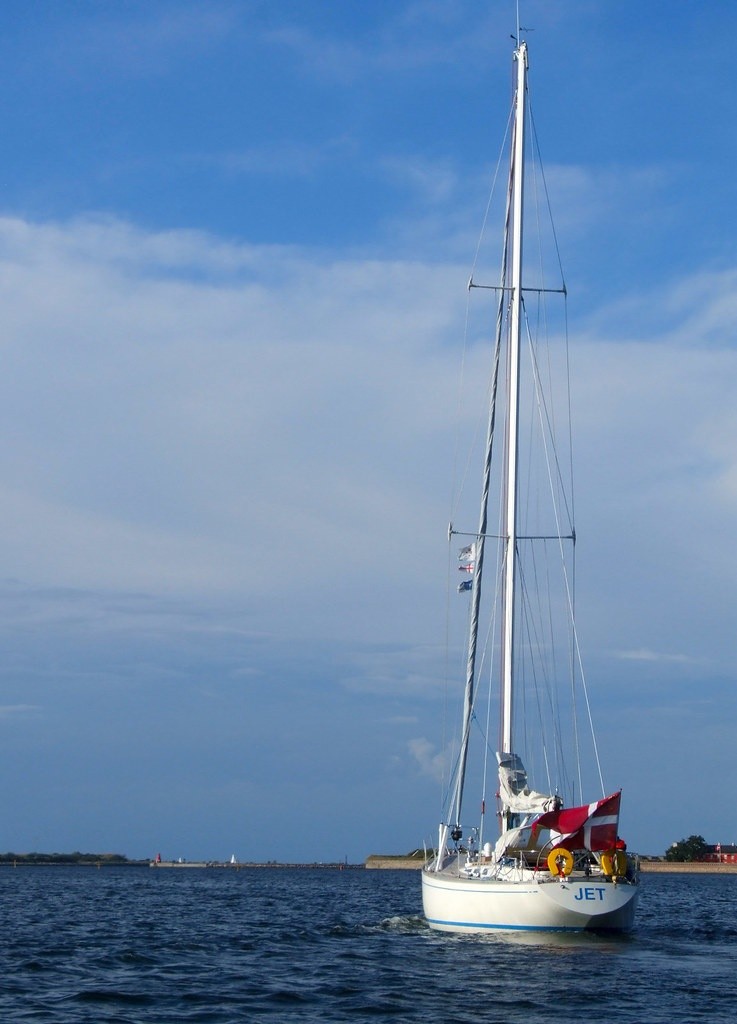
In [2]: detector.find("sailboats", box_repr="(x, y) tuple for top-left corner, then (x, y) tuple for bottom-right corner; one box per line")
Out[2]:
(421, 30), (644, 941)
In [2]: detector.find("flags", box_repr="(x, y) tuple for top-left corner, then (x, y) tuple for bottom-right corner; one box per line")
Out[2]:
(456, 542), (476, 594)
(715, 845), (721, 851)
(531, 791), (621, 874)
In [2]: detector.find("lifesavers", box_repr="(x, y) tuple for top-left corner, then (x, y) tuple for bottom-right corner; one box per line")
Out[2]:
(548, 848), (574, 876)
(601, 849), (627, 876)
